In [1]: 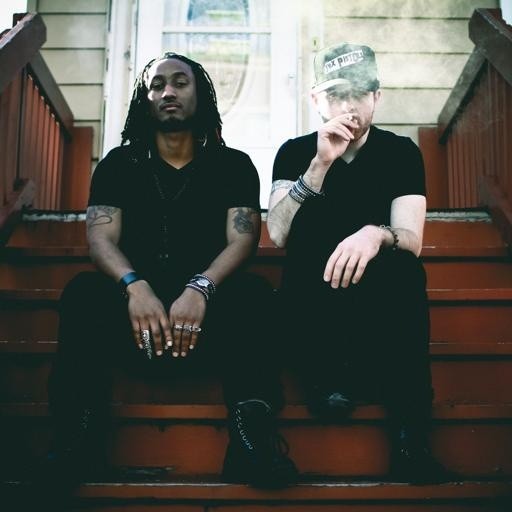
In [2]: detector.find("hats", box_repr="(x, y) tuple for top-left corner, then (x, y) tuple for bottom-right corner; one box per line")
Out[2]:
(311, 44), (383, 96)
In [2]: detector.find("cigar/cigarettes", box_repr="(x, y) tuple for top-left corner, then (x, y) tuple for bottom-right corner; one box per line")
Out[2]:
(348, 116), (354, 121)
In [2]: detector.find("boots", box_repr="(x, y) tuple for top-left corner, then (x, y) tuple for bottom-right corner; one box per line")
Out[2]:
(30, 401), (111, 484)
(221, 401), (297, 487)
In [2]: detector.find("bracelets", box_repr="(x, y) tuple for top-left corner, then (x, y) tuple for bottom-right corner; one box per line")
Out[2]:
(288, 175), (326, 205)
(118, 271), (151, 302)
(379, 224), (399, 251)
(185, 273), (217, 302)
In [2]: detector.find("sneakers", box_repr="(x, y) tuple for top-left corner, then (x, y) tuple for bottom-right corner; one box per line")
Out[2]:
(304, 384), (356, 413)
(385, 439), (449, 482)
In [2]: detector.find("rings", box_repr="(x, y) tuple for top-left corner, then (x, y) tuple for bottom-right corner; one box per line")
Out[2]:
(183, 325), (192, 331)
(192, 327), (202, 334)
(173, 324), (183, 330)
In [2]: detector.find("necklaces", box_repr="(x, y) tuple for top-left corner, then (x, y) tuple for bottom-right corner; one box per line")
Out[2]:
(152, 173), (187, 259)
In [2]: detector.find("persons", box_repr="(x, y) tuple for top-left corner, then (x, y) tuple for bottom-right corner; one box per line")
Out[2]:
(38, 51), (300, 485)
(267, 41), (466, 485)
